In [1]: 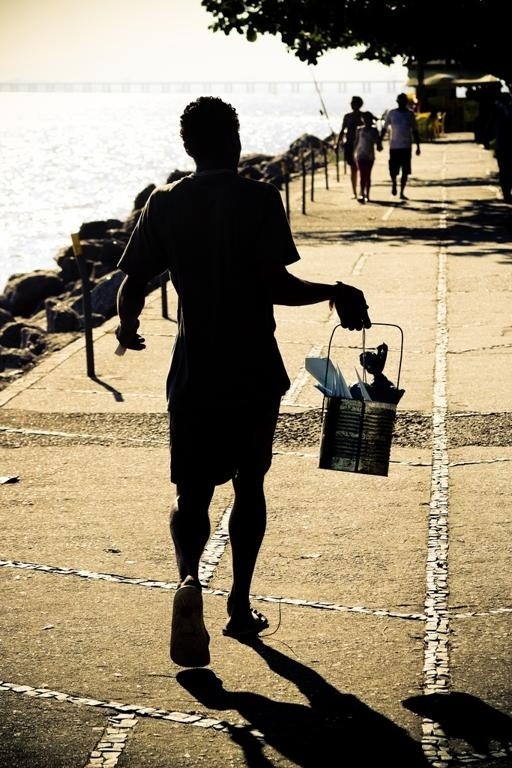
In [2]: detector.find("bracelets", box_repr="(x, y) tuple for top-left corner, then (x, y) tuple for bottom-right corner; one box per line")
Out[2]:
(329, 281), (342, 311)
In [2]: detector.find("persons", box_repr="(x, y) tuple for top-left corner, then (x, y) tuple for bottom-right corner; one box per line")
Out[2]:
(114, 95), (370, 668)
(353, 111), (383, 202)
(380, 93), (420, 200)
(334, 96), (365, 199)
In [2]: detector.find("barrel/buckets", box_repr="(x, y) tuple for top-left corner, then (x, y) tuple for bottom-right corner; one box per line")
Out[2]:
(317, 323), (405, 478)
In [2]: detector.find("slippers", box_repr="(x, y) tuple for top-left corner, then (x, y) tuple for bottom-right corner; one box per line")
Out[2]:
(170, 585), (211, 667)
(221, 610), (269, 637)
